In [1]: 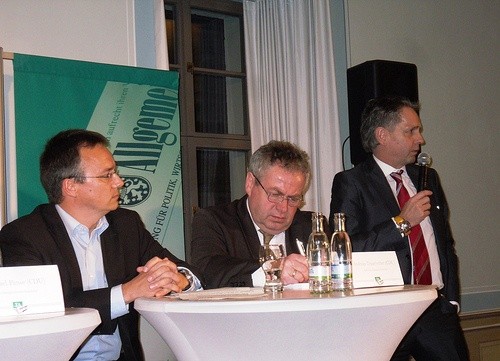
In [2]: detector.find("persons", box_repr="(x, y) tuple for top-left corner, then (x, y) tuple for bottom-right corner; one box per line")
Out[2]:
(192, 140), (328, 287)
(0, 129), (204, 361)
(330, 96), (472, 361)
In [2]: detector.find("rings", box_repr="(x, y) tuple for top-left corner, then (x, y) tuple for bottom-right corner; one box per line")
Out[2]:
(292, 269), (297, 277)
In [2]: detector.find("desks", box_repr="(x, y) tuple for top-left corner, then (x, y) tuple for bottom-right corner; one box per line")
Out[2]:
(135, 284), (439, 361)
(0, 308), (102, 361)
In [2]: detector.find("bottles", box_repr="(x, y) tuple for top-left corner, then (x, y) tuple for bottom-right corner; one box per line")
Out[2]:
(306, 211), (331, 296)
(331, 212), (353, 293)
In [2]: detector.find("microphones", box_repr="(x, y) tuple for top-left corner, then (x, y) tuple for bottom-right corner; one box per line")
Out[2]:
(417, 152), (431, 194)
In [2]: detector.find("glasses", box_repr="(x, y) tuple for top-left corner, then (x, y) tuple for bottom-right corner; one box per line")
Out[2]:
(252, 174), (304, 207)
(63, 171), (120, 183)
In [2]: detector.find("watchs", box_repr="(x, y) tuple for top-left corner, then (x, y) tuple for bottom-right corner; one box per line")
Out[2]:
(178, 269), (193, 291)
(394, 216), (411, 237)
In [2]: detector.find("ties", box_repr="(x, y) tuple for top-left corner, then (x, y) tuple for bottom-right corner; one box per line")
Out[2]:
(258, 229), (278, 278)
(390, 170), (432, 286)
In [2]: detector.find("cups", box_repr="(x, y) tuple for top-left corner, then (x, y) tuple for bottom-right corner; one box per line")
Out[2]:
(259, 244), (285, 294)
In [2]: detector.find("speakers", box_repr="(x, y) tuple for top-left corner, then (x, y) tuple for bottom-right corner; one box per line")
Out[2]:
(347, 59), (420, 166)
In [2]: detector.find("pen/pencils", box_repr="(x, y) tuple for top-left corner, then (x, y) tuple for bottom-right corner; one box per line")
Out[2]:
(297, 238), (305, 257)
